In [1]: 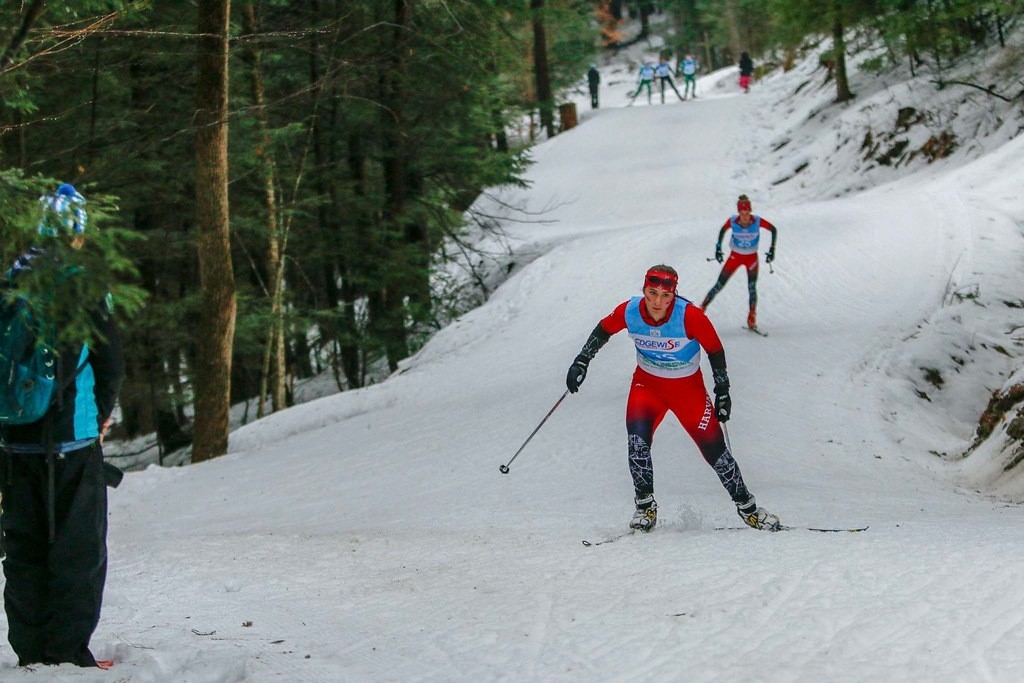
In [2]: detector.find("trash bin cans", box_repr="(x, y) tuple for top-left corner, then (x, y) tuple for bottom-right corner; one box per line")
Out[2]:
(558, 103), (578, 131)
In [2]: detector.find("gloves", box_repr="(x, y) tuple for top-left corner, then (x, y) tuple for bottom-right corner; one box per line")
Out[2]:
(714, 243), (724, 263)
(713, 385), (731, 423)
(566, 355), (588, 394)
(766, 248), (774, 263)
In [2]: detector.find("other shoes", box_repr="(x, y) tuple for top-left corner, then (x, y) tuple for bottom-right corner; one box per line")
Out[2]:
(747, 315), (757, 328)
(94, 660), (114, 670)
(700, 304), (706, 313)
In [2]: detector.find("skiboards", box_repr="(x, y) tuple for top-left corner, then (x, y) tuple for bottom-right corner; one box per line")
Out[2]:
(741, 326), (770, 338)
(581, 524), (872, 546)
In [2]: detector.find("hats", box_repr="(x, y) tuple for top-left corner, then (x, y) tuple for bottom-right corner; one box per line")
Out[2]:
(737, 195), (751, 212)
(38, 183), (88, 240)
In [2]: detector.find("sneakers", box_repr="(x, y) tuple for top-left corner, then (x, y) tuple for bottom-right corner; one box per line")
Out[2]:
(737, 496), (779, 533)
(629, 496), (658, 531)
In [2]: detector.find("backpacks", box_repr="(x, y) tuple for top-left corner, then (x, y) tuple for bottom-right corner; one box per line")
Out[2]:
(0, 266), (89, 430)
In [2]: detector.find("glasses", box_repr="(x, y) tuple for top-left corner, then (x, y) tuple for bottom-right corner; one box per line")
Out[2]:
(645, 275), (677, 286)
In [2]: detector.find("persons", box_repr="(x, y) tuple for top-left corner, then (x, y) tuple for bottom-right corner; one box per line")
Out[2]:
(0, 182), (124, 669)
(738, 51), (753, 93)
(588, 64), (599, 108)
(655, 57), (684, 104)
(566, 264), (781, 532)
(697, 195), (776, 328)
(631, 61), (656, 103)
(676, 54), (700, 99)
